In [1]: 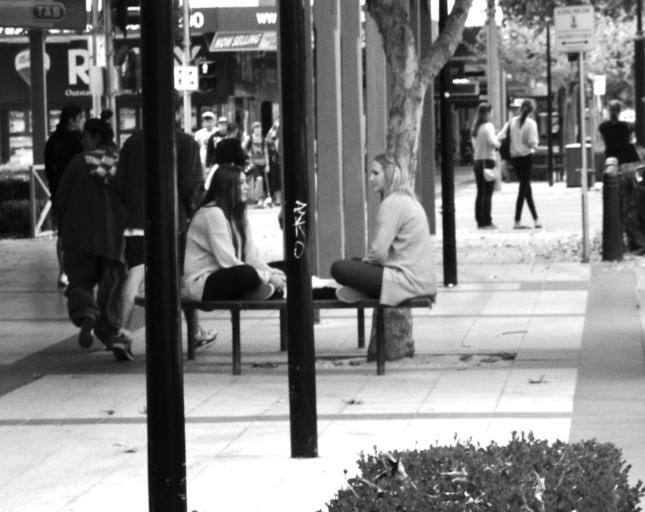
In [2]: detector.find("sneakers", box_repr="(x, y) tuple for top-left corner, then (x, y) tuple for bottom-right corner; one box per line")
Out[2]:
(335, 286), (365, 304)
(58, 271), (218, 361)
(252, 283), (276, 299)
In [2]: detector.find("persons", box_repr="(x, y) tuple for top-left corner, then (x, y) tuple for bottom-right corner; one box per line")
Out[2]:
(52, 118), (127, 351)
(181, 164), (288, 301)
(101, 109), (113, 124)
(111, 89), (217, 361)
(497, 99), (544, 230)
(330, 151), (437, 307)
(468, 101), (502, 230)
(43, 105), (84, 286)
(597, 99), (640, 164)
(193, 112), (283, 209)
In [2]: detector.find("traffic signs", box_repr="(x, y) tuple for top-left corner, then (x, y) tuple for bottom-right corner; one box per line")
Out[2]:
(553, 6), (595, 53)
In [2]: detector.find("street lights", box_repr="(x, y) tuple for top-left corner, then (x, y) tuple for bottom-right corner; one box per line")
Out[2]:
(592, 75), (607, 186)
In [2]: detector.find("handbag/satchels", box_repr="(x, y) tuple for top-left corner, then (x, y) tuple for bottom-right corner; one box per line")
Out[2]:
(499, 129), (510, 160)
(483, 166), (499, 182)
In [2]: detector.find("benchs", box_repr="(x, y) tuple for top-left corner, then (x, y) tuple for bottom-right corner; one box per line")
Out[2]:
(134, 292), (434, 376)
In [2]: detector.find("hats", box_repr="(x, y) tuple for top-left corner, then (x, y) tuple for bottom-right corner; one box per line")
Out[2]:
(202, 111), (217, 123)
